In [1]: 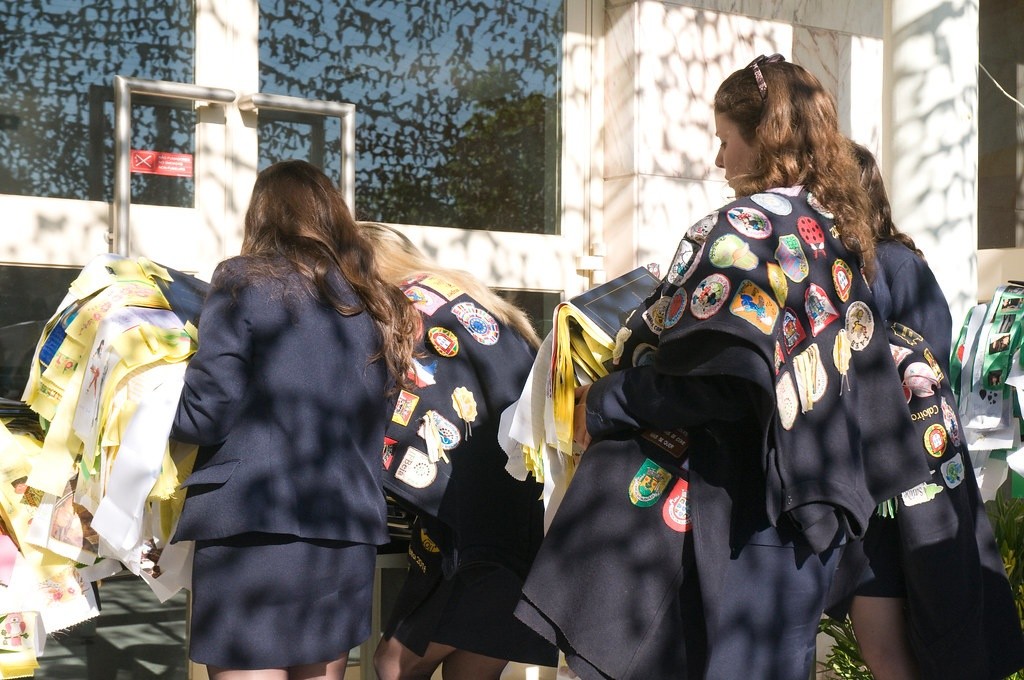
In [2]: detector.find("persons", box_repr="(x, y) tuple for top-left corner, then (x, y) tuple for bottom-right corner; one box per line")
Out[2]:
(511, 51), (933, 680)
(352, 221), (560, 680)
(822, 136), (1024, 680)
(166, 159), (428, 680)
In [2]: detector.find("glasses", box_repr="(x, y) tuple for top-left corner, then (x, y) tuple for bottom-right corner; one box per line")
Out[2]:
(745, 52), (785, 101)
